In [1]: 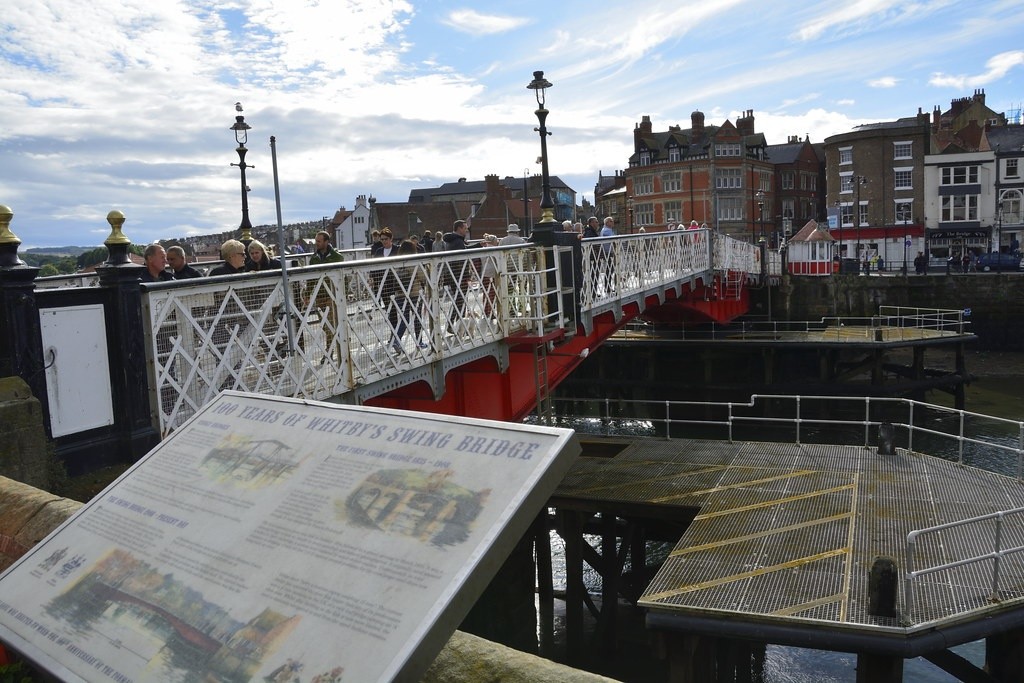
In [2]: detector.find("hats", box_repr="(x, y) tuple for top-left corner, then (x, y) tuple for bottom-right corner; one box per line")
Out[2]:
(506, 224), (521, 233)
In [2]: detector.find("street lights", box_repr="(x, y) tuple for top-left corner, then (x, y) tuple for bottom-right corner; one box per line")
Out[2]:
(527, 70), (554, 222)
(900, 204), (908, 275)
(834, 199), (843, 273)
(998, 200), (1003, 273)
(230, 114), (255, 246)
(756, 188), (764, 238)
(848, 174), (868, 258)
(628, 195), (635, 233)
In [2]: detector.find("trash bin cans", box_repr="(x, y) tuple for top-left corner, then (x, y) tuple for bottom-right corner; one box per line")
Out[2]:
(878, 423), (895, 454)
(875, 328), (882, 341)
(869, 557), (898, 617)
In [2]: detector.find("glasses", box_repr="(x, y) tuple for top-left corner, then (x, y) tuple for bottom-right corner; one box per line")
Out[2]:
(236, 252), (246, 257)
(380, 237), (389, 242)
(591, 221), (598, 224)
(610, 221), (614, 222)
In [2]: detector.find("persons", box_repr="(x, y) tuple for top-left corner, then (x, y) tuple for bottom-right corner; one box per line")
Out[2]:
(303, 230), (345, 363)
(950, 250), (976, 273)
(914, 252), (928, 275)
(368, 219), (527, 354)
(877, 255), (883, 276)
(671, 220), (708, 271)
(561, 217), (616, 299)
(140, 238), (284, 412)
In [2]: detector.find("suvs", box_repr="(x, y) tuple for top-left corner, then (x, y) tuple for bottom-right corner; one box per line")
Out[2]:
(976, 253), (1021, 272)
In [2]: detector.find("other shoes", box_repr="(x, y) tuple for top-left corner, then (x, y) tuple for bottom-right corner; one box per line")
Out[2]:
(321, 349), (334, 364)
(592, 293), (601, 298)
(446, 327), (454, 334)
(392, 340), (404, 353)
(416, 342), (427, 349)
